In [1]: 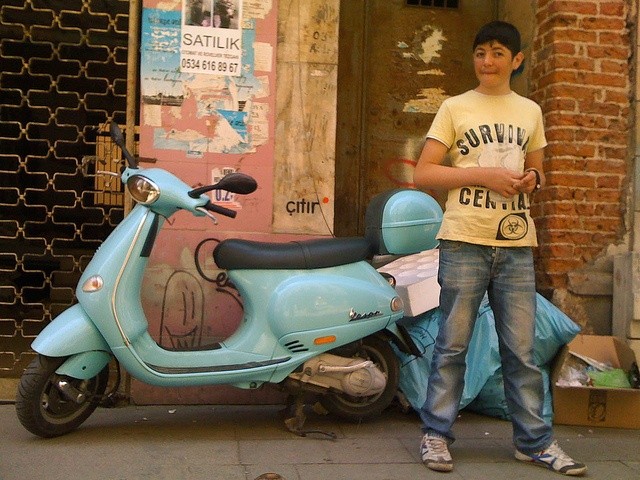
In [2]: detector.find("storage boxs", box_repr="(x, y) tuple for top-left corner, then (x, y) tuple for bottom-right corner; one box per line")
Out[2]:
(551, 335), (639, 429)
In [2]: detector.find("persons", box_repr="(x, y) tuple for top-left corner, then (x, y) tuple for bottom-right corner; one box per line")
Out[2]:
(412, 20), (587, 475)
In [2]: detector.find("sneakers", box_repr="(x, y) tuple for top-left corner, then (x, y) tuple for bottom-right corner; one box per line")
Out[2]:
(420, 433), (453, 471)
(515, 439), (586, 475)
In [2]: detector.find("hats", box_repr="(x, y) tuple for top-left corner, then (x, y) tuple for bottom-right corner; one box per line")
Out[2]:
(473, 21), (524, 75)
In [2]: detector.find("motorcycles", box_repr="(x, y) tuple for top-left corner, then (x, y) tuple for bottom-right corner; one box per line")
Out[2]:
(15, 116), (444, 439)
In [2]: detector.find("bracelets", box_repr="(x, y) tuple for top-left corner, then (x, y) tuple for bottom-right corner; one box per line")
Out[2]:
(527, 170), (541, 193)
